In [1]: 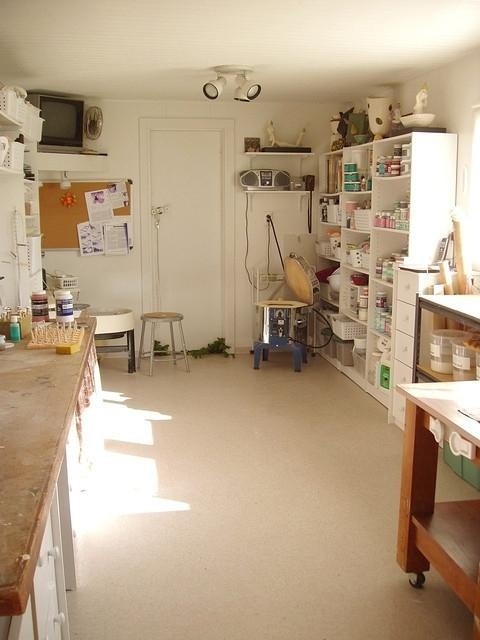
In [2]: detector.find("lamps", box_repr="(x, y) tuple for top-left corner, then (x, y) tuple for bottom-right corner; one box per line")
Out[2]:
(202, 65), (261, 101)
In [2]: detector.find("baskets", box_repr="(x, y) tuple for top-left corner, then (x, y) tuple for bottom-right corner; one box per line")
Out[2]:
(46, 274), (78, 289)
(26, 232), (43, 277)
(0, 89), (45, 142)
(2, 141), (25, 171)
(330, 313), (367, 341)
(352, 209), (370, 231)
(315, 242), (334, 257)
(346, 248), (369, 270)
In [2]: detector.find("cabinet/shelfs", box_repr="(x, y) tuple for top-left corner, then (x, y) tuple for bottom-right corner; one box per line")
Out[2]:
(1, 113), (42, 318)
(390, 267), (479, 451)
(0, 333), (101, 639)
(314, 133), (457, 411)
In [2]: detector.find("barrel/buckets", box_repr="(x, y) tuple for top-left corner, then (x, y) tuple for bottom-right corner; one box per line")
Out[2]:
(375, 348), (392, 402)
(430, 329), (468, 375)
(450, 340), (475, 382)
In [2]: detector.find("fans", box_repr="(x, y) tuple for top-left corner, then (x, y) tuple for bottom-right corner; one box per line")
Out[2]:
(84, 106), (103, 140)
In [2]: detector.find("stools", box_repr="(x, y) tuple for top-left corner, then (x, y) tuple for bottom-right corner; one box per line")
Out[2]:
(85, 307), (191, 377)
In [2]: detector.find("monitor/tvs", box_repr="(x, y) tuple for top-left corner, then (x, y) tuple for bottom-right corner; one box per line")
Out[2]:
(27, 89), (84, 143)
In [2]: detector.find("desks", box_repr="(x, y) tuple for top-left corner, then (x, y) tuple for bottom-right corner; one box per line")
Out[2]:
(396, 380), (480, 639)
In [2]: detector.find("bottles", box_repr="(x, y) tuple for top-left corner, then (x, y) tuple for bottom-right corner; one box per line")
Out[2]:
(319, 197), (339, 224)
(375, 246), (407, 285)
(30, 291), (50, 322)
(374, 200), (409, 231)
(345, 201), (370, 230)
(358, 287), (369, 321)
(373, 291), (391, 337)
(54, 290), (75, 323)
(9, 315), (21, 344)
(361, 175), (371, 191)
(377, 142), (401, 176)
(368, 351), (382, 384)
(290, 176), (306, 190)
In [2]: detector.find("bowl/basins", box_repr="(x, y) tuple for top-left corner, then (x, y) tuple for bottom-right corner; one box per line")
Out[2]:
(316, 265), (339, 292)
(353, 134), (369, 144)
(351, 274), (368, 286)
(402, 113), (434, 127)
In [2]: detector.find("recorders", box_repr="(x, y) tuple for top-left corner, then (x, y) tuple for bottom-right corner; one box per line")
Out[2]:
(239, 170), (291, 190)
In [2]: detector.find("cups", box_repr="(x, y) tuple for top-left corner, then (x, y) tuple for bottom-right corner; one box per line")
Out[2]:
(401, 143), (410, 176)
(429, 329), (480, 381)
